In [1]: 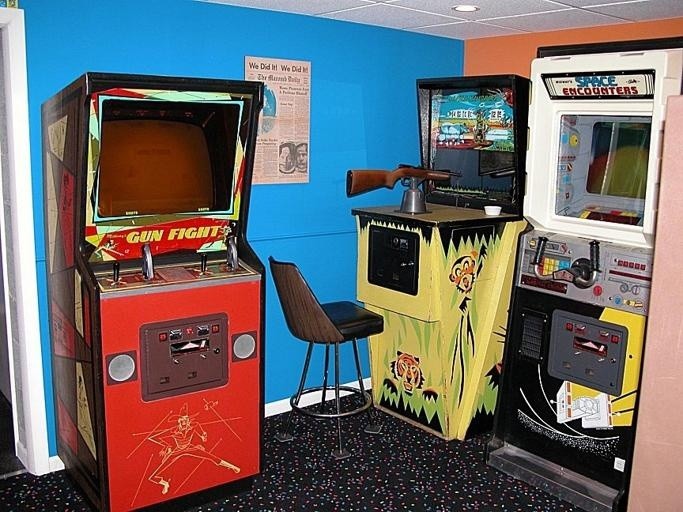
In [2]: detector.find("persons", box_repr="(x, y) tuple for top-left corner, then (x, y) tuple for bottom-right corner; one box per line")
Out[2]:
(295, 143), (306, 173)
(278, 143), (295, 174)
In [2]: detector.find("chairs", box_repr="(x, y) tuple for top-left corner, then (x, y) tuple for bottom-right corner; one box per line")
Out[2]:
(266, 254), (383, 462)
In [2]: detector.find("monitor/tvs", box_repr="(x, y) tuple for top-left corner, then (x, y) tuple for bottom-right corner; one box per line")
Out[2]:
(97, 117), (217, 218)
(429, 89), (517, 204)
(584, 120), (652, 202)
